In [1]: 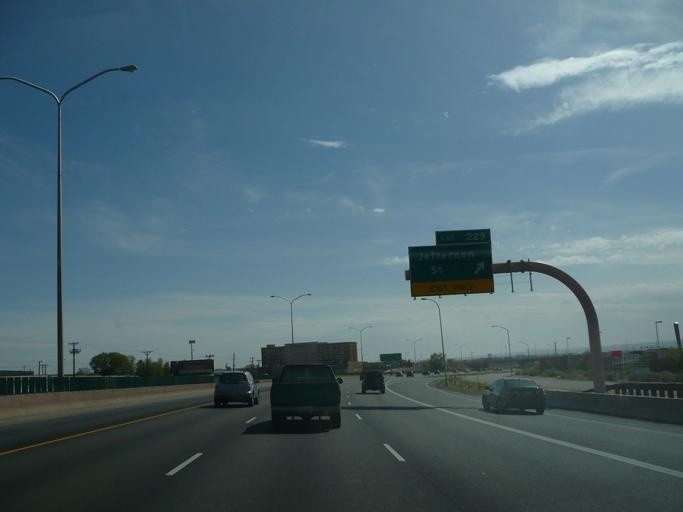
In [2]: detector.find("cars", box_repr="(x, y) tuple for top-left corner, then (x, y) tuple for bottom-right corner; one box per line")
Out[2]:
(396, 368), (440, 378)
(482, 378), (546, 414)
(454, 368), (503, 373)
(214, 371), (260, 408)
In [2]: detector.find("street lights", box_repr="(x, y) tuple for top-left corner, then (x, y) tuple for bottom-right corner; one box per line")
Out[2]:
(567, 337), (571, 354)
(516, 341), (529, 369)
(406, 338), (423, 369)
(492, 325), (513, 376)
(655, 321), (663, 346)
(348, 325), (373, 368)
(421, 298), (447, 387)
(0, 66), (138, 390)
(270, 294), (311, 343)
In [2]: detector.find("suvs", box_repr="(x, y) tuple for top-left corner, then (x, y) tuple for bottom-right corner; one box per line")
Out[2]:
(361, 370), (385, 394)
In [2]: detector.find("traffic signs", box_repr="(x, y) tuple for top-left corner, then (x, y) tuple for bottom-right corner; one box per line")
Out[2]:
(408, 228), (494, 296)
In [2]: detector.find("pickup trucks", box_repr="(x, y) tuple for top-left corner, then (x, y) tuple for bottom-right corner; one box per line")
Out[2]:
(270, 363), (344, 429)
